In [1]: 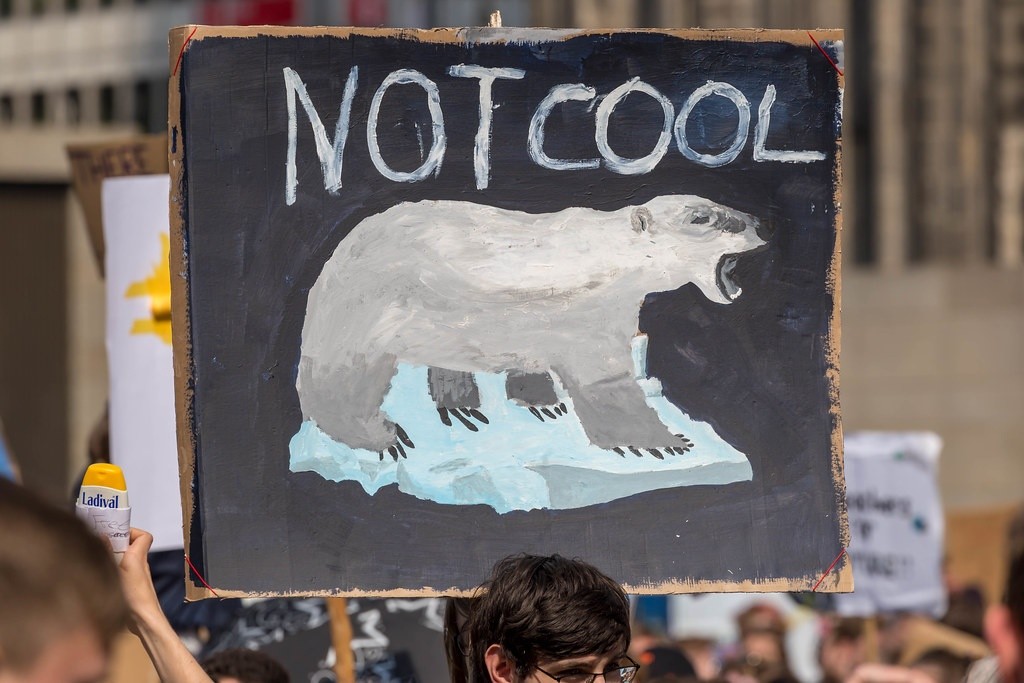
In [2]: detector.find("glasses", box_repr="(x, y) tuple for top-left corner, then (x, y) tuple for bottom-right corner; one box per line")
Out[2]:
(532, 656), (640, 683)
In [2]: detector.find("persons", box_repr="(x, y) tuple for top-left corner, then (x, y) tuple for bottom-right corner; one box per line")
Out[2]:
(457, 551), (641, 682)
(0, 479), (127, 683)
(631, 510), (1024, 683)
